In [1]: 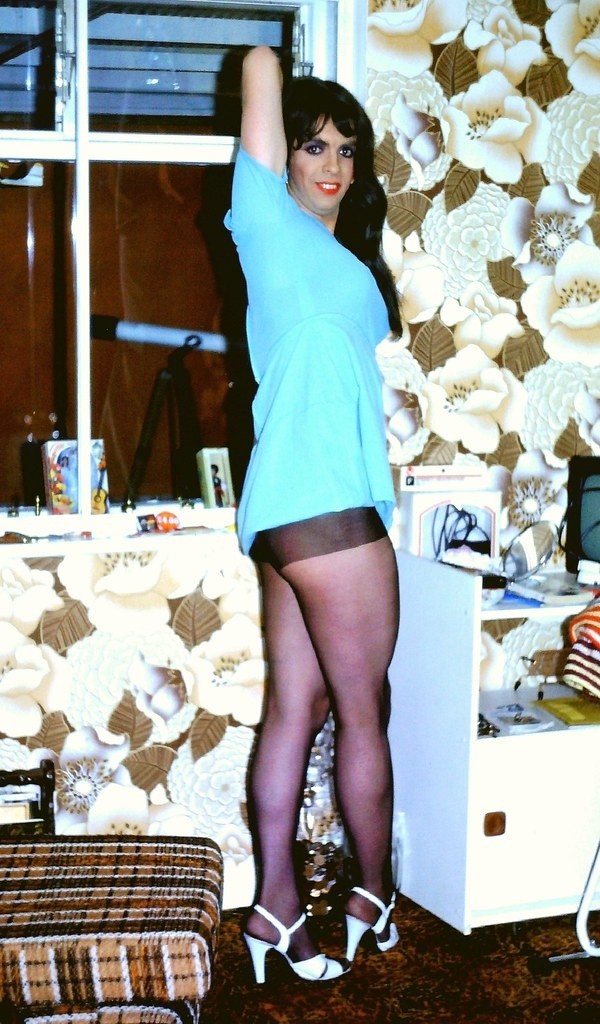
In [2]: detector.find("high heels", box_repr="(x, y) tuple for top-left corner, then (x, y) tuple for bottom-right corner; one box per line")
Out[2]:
(241, 904), (349, 986)
(341, 887), (402, 959)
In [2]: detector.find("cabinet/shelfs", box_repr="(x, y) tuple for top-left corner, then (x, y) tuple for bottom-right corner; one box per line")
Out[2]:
(388, 542), (599, 933)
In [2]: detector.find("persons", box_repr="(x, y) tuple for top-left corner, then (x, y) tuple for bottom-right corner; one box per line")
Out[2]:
(221, 42), (405, 981)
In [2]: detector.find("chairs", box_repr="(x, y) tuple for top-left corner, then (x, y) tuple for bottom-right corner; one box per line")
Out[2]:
(0, 832), (229, 1023)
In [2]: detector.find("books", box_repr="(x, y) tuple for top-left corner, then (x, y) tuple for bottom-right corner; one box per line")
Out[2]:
(508, 578), (595, 604)
